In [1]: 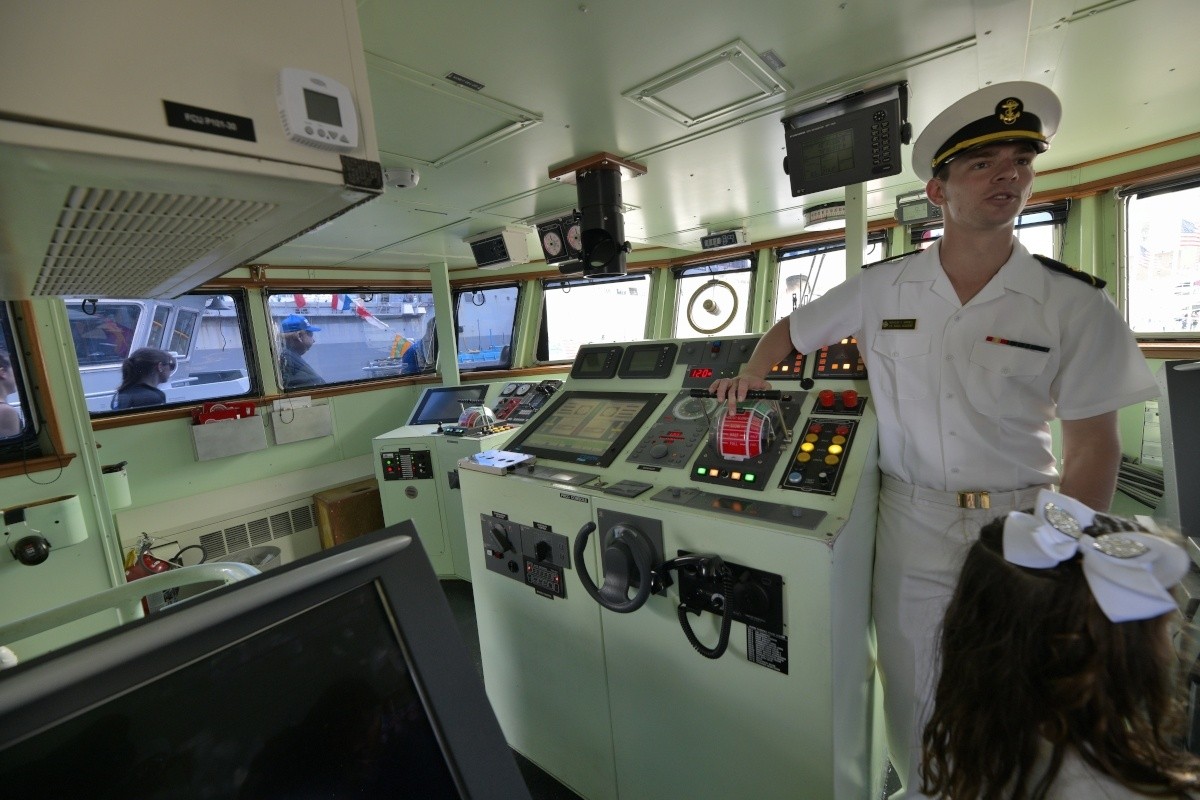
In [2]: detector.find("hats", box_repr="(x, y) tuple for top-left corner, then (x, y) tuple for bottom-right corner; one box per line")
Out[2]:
(280, 315), (320, 333)
(911, 81), (1063, 184)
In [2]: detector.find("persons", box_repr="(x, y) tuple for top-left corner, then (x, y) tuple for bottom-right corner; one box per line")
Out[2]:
(918, 488), (1200, 800)
(117, 347), (175, 408)
(402, 317), (436, 374)
(278, 314), (327, 389)
(0, 351), (24, 440)
(710, 80), (1162, 800)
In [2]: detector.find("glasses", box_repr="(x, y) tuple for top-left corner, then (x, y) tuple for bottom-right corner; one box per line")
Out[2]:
(162, 362), (175, 370)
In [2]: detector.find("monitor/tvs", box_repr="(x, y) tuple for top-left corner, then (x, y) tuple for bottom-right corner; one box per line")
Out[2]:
(410, 384), (489, 425)
(0, 517), (532, 800)
(504, 392), (667, 468)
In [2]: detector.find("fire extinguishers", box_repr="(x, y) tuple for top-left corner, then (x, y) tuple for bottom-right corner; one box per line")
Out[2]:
(124, 531), (208, 582)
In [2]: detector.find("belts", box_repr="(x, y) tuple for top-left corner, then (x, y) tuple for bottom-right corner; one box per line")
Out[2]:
(883, 475), (1053, 510)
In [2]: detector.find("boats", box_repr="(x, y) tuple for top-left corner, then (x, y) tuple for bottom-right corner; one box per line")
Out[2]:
(0, 249), (847, 424)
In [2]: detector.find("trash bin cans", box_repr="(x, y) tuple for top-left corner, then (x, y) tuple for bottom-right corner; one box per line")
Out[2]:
(214, 545), (282, 574)
